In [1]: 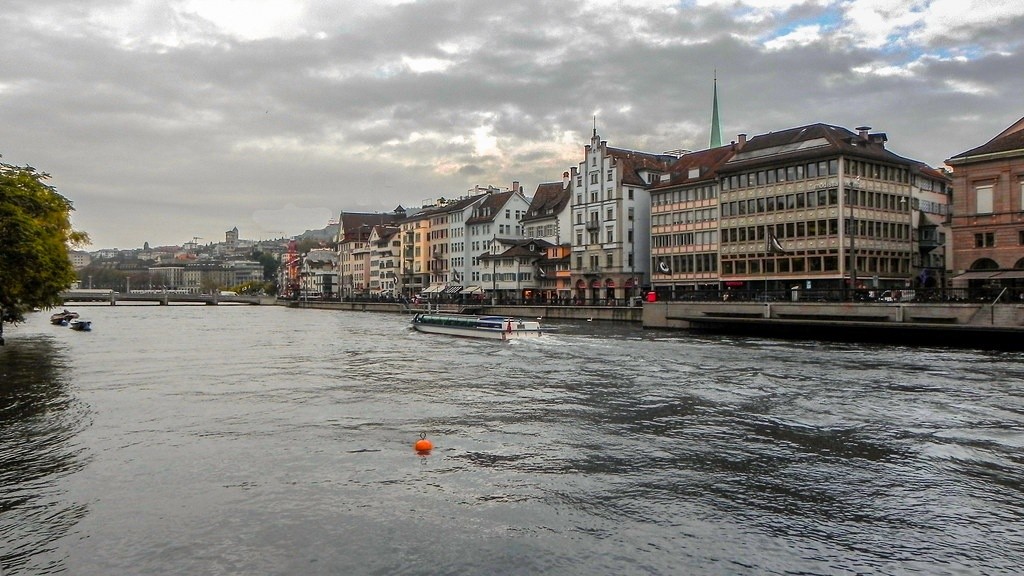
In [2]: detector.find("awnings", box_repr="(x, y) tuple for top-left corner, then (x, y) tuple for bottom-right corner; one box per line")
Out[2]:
(423, 285), (482, 295)
(949, 270), (1024, 279)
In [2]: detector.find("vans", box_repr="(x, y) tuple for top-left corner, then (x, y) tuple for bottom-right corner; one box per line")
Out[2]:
(220, 291), (239, 297)
(877, 289), (916, 302)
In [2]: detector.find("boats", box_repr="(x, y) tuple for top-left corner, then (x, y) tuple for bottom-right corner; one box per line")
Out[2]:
(407, 311), (559, 340)
(32, 307), (42, 312)
(70, 318), (92, 331)
(49, 312), (80, 325)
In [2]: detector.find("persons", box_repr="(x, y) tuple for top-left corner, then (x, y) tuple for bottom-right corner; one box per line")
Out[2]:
(553, 294), (581, 308)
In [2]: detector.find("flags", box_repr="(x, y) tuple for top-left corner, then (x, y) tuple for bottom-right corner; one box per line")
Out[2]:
(656, 253), (672, 276)
(767, 228), (788, 255)
